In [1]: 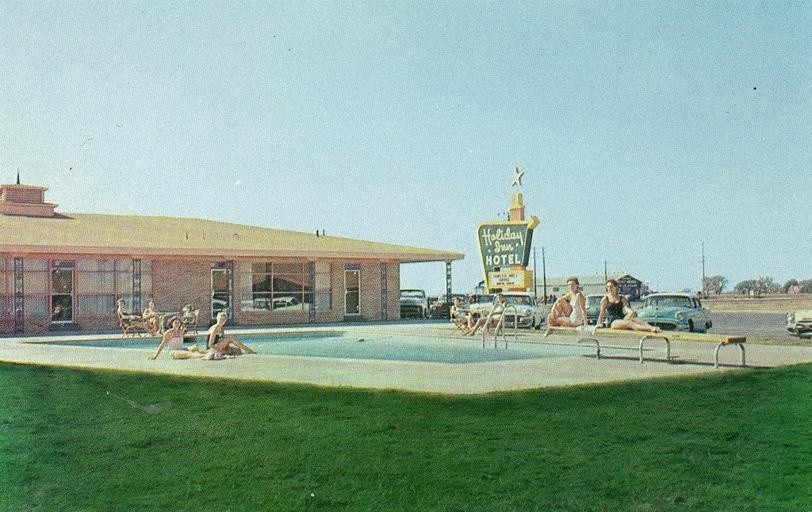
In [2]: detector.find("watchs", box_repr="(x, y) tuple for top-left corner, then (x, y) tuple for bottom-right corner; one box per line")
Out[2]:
(116, 299), (144, 325)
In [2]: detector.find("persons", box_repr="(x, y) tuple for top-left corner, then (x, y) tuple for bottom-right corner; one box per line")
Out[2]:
(143, 299), (160, 332)
(450, 297), (475, 330)
(464, 294), (507, 336)
(148, 316), (205, 360)
(544, 276), (588, 339)
(537, 293), (557, 304)
(165, 305), (197, 334)
(207, 311), (258, 355)
(596, 278), (662, 334)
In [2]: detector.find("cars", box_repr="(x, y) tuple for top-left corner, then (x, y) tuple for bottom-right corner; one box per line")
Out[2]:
(400, 288), (542, 329)
(638, 293), (712, 333)
(213, 299), (228, 315)
(786, 310), (812, 338)
(586, 294), (604, 325)
(255, 297), (309, 311)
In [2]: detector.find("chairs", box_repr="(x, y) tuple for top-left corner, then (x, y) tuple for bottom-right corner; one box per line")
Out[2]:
(115, 309), (201, 339)
(449, 306), (502, 336)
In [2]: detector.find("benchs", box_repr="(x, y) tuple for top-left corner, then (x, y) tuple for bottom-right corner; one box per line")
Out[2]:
(547, 327), (747, 368)
